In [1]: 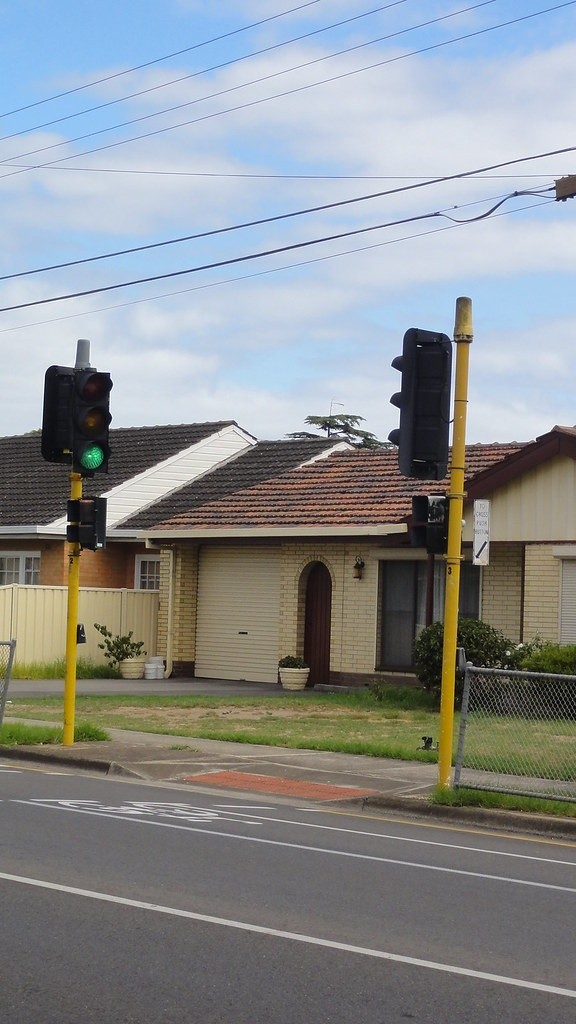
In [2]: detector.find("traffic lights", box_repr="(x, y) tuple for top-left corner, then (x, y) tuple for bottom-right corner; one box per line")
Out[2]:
(71, 370), (114, 478)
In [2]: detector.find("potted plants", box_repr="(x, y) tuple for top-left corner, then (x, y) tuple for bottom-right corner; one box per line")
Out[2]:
(275, 653), (311, 690)
(96, 623), (147, 680)
